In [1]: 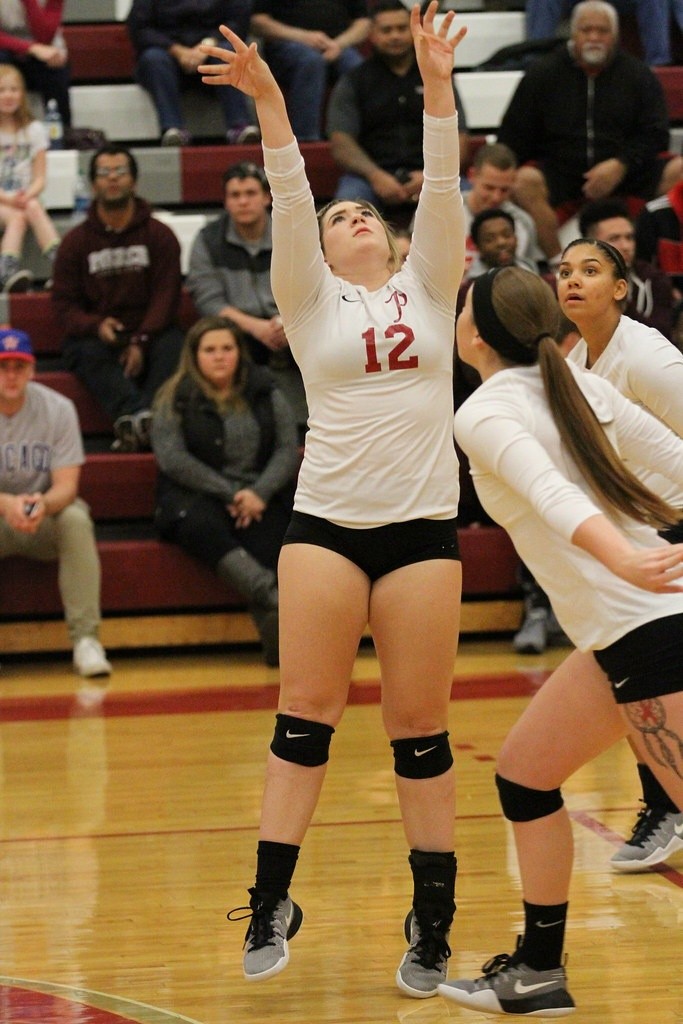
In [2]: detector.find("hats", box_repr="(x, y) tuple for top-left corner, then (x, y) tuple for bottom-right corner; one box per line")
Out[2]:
(0, 324), (34, 362)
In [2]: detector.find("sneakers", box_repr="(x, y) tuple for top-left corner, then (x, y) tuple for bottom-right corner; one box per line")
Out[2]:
(74, 638), (111, 679)
(438, 936), (575, 1018)
(397, 903), (456, 999)
(607, 799), (683, 871)
(227, 892), (304, 982)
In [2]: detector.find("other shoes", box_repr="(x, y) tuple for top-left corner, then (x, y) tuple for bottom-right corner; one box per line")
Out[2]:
(162, 127), (190, 147)
(131, 411), (153, 439)
(45, 279), (54, 289)
(259, 626), (280, 667)
(5, 269), (35, 295)
(512, 606), (548, 654)
(228, 124), (260, 144)
(112, 415), (146, 453)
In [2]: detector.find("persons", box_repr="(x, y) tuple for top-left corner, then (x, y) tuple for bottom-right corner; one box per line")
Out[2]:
(151, 316), (299, 671)
(436, 261), (683, 1016)
(182, 162), (301, 380)
(47, 143), (188, 451)
(447, 140), (683, 651)
(0, 63), (63, 289)
(557, 238), (683, 870)
(0, 0), (104, 151)
(196, 1), (466, 1000)
(1, 327), (115, 680)
(125, 0), (681, 225)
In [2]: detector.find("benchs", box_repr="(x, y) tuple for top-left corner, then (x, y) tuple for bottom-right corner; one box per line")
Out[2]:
(0, 1), (682, 618)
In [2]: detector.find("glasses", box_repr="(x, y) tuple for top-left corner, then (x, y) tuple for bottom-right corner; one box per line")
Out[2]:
(94, 165), (128, 176)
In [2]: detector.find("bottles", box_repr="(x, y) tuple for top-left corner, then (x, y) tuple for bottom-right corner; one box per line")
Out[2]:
(72, 176), (91, 223)
(44, 99), (64, 149)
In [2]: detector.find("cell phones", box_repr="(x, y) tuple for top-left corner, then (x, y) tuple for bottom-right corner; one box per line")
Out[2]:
(115, 325), (138, 335)
